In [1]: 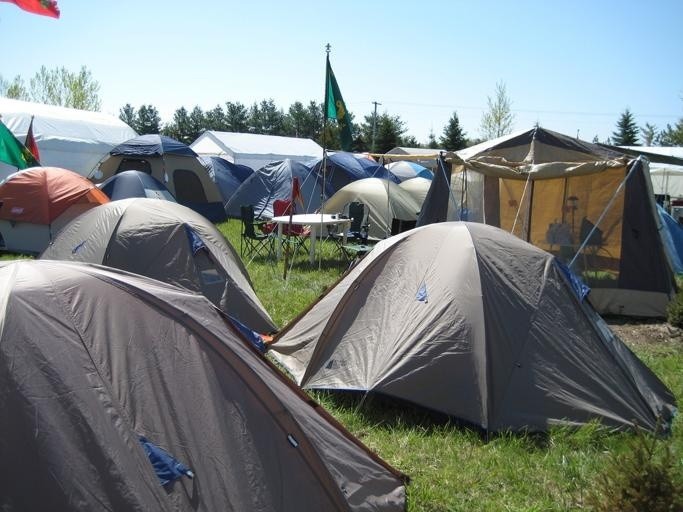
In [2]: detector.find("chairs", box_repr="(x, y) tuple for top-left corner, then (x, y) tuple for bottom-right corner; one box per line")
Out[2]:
(328, 202), (370, 255)
(239, 205), (279, 258)
(262, 201), (311, 257)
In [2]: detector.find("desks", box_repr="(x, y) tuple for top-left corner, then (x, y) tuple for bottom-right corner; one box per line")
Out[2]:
(271, 212), (353, 265)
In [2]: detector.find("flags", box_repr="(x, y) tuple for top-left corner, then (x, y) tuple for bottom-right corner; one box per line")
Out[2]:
(2, 1), (63, 20)
(322, 60), (357, 149)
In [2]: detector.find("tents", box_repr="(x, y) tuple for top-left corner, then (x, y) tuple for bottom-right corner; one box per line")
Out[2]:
(264, 220), (678, 443)
(0, 258), (413, 512)
(417, 123), (682, 323)
(1, 101), (433, 344)
